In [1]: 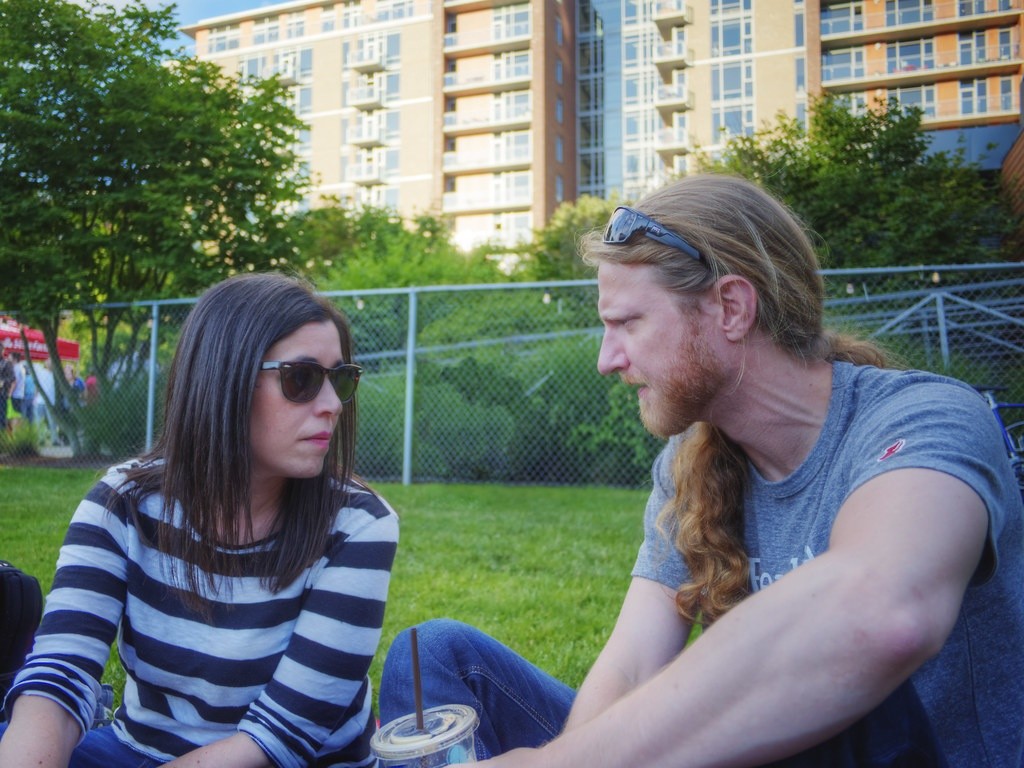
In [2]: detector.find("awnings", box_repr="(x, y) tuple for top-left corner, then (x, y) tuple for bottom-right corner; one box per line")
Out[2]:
(0, 324), (79, 361)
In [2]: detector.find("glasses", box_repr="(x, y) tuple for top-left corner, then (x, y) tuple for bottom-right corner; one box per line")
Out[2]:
(605, 206), (713, 267)
(258, 360), (364, 402)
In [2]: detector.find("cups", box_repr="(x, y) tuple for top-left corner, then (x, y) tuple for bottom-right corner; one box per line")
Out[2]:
(370, 703), (480, 768)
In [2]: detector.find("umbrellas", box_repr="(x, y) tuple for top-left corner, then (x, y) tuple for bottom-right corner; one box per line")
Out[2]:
(107, 351), (162, 377)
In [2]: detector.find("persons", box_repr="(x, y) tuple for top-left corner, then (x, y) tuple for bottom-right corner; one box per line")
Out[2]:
(0, 350), (98, 444)
(377, 173), (1024, 768)
(0, 273), (399, 768)
